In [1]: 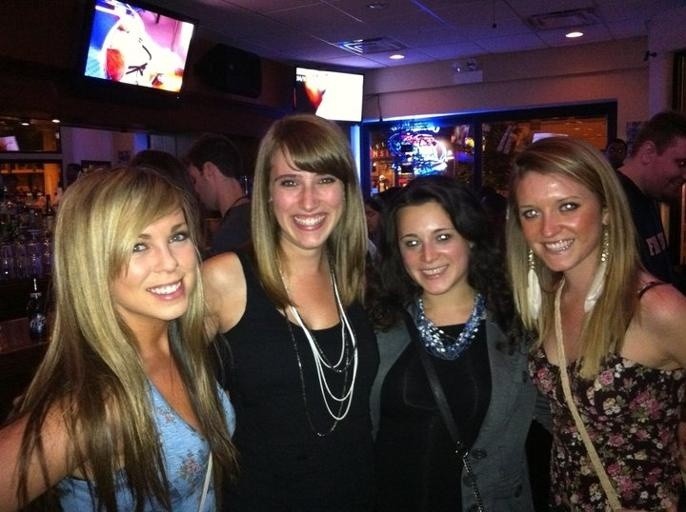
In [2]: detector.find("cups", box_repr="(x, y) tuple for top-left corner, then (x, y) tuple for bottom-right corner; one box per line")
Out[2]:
(0, 229), (53, 280)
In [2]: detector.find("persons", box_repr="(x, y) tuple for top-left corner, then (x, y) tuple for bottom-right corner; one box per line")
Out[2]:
(197, 113), (380, 512)
(362, 111), (686, 512)
(129, 150), (196, 199)
(0, 164), (236, 512)
(183, 132), (252, 249)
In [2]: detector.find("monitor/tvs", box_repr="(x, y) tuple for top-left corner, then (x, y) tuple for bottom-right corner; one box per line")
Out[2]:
(81, 0), (198, 99)
(291, 65), (365, 122)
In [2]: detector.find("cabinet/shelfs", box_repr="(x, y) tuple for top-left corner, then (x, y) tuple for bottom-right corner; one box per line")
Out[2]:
(0, 75), (65, 321)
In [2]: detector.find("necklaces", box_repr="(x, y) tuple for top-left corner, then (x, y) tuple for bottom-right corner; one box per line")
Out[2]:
(224, 196), (248, 218)
(272, 263), (360, 440)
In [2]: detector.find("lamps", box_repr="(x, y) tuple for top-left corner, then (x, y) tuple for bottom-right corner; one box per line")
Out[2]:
(451, 58), (483, 85)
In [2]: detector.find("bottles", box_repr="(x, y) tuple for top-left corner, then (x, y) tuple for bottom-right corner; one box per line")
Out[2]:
(0, 164), (58, 241)
(26, 275), (49, 339)
